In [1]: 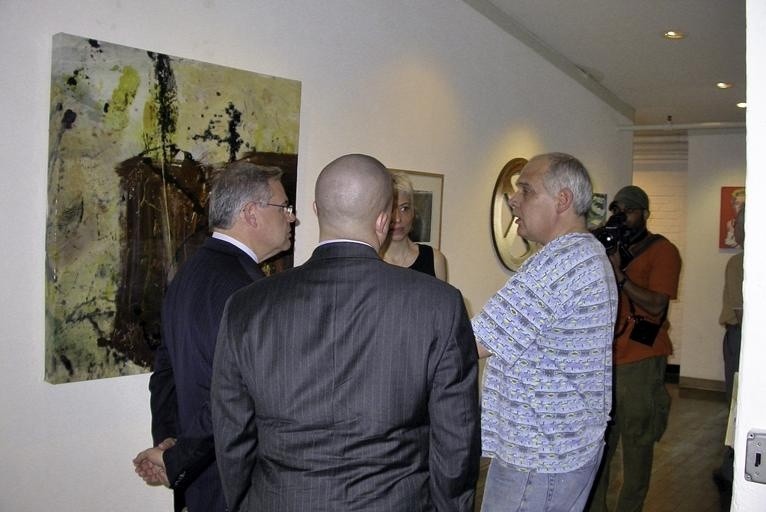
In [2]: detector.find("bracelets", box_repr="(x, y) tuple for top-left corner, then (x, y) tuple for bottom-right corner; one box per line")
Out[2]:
(618, 277), (629, 289)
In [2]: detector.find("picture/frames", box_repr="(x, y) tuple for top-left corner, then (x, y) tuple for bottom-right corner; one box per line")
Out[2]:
(387, 168), (445, 251)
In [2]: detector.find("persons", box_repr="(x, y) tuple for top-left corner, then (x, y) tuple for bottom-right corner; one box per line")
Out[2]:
(710, 205), (745, 489)
(469, 152), (620, 512)
(586, 184), (683, 510)
(132, 161), (297, 511)
(208, 152), (481, 512)
(376, 168), (447, 286)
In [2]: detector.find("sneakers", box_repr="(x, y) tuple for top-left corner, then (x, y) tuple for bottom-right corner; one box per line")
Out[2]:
(713, 469), (731, 493)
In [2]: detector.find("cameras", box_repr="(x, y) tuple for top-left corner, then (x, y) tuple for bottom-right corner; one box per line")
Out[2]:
(591, 210), (628, 256)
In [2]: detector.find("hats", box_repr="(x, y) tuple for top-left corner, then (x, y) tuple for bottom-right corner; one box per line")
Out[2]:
(609, 186), (648, 211)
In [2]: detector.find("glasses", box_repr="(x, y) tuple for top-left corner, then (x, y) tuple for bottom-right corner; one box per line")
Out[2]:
(243, 200), (294, 219)
(611, 205), (635, 214)
